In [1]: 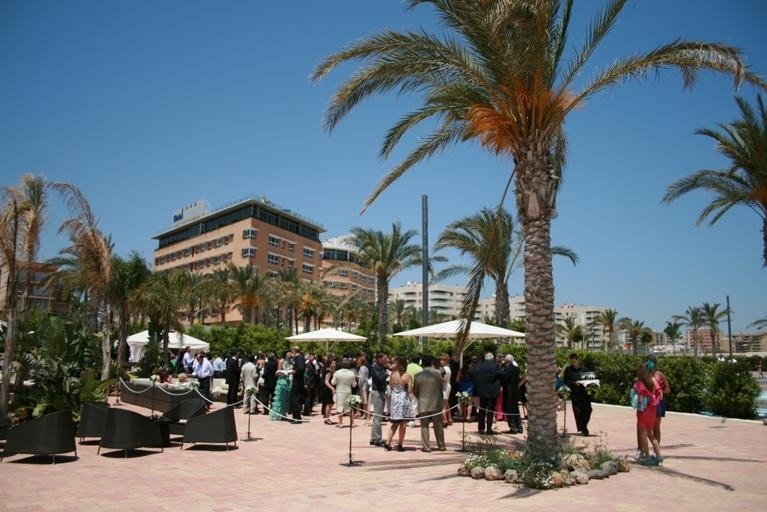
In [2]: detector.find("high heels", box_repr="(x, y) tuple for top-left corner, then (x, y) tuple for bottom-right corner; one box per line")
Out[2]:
(384, 444), (405, 452)
(324, 420), (335, 425)
(652, 456), (664, 466)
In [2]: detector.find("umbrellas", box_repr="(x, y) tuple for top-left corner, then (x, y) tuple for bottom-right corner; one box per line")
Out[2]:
(285, 328), (368, 359)
(392, 318), (525, 370)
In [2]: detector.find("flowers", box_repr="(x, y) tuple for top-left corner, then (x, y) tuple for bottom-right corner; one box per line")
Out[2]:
(558, 383), (571, 400)
(243, 384), (258, 400)
(453, 389), (471, 405)
(344, 392), (362, 414)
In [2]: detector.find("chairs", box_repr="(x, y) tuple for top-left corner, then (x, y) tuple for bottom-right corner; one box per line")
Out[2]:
(158, 399), (207, 433)
(178, 405), (236, 453)
(78, 400), (110, 442)
(0, 408), (77, 467)
(96, 405), (170, 460)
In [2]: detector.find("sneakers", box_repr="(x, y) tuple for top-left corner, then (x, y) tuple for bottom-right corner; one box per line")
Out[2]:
(508, 427), (523, 434)
(370, 439), (386, 447)
(479, 429), (498, 435)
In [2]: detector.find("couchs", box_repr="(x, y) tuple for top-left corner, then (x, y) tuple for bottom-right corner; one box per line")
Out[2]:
(117, 376), (231, 414)
